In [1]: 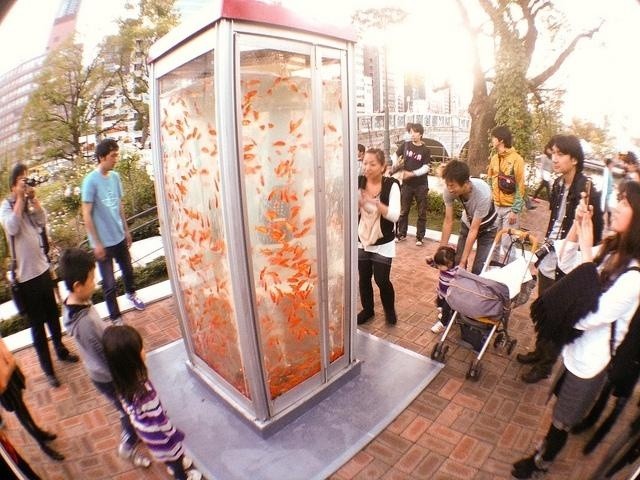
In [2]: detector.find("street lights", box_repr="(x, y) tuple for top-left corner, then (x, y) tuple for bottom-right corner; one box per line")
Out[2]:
(375, 26), (394, 168)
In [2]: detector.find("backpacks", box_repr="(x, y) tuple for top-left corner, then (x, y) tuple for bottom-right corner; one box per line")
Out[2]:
(606, 268), (640, 400)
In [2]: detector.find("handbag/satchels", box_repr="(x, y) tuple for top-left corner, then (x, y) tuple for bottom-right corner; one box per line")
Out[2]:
(490, 240), (535, 309)
(497, 171), (517, 194)
(460, 195), (498, 232)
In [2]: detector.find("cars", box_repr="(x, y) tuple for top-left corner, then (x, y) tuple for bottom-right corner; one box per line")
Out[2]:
(27, 141), (153, 209)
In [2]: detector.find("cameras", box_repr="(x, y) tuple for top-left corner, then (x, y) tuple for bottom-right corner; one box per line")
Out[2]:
(535, 239), (553, 260)
(25, 178), (36, 185)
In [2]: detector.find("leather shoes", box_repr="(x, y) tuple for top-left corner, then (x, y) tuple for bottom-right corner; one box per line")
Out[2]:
(517, 354), (535, 366)
(356, 309), (375, 325)
(511, 463), (548, 479)
(513, 451), (540, 469)
(384, 308), (398, 327)
(46, 373), (59, 387)
(40, 432), (57, 442)
(48, 452), (65, 462)
(523, 367), (550, 383)
(60, 353), (79, 363)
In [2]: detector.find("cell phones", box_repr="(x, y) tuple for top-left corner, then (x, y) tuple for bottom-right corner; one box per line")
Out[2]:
(585, 181), (592, 204)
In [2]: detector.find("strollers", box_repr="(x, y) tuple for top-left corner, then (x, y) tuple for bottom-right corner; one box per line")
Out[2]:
(430, 228), (538, 382)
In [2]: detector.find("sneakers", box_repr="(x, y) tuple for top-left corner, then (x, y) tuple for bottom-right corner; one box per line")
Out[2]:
(431, 320), (448, 334)
(436, 307), (444, 320)
(183, 470), (203, 480)
(126, 292), (145, 310)
(114, 316), (125, 327)
(167, 459), (193, 476)
(117, 445), (151, 469)
(394, 234), (407, 243)
(415, 239), (424, 246)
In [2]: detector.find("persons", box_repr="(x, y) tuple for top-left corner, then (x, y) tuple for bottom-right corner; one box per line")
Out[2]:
(0, 331), (65, 462)
(60, 248), (151, 472)
(1, 162), (78, 387)
(80, 139), (145, 327)
(103, 324), (201, 480)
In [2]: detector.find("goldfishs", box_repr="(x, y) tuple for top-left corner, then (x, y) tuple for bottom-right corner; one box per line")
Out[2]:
(159, 70), (345, 400)
